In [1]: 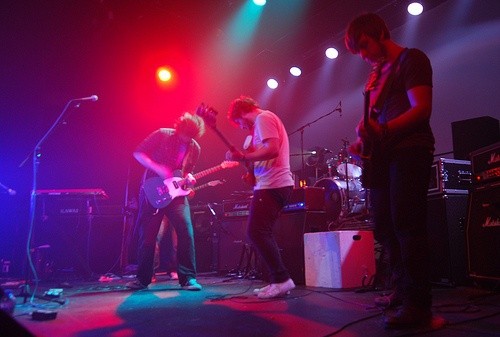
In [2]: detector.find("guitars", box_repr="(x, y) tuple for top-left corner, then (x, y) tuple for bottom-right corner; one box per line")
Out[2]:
(336, 145), (363, 178)
(315, 177), (366, 218)
(360, 68), (387, 190)
(192, 179), (226, 192)
(142, 161), (240, 209)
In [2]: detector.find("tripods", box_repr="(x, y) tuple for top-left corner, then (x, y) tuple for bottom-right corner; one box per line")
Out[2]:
(14, 100), (72, 304)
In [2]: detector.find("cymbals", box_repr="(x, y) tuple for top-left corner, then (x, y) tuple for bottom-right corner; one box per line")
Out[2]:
(310, 146), (332, 153)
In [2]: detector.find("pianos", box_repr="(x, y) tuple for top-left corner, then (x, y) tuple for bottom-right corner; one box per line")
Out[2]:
(221, 184), (326, 280)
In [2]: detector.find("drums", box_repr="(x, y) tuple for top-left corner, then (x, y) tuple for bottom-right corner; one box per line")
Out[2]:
(326, 157), (344, 180)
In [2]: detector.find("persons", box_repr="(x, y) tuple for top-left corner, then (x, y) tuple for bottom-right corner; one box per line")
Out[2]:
(120, 162), (168, 276)
(227, 97), (294, 299)
(131, 112), (205, 292)
(344, 11), (435, 330)
(151, 189), (197, 283)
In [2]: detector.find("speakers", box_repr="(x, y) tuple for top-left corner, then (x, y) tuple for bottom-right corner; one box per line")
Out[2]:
(191, 209), (328, 285)
(31, 212), (133, 277)
(426, 194), (469, 286)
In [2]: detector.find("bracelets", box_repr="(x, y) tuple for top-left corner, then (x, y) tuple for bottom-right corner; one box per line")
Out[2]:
(377, 119), (388, 140)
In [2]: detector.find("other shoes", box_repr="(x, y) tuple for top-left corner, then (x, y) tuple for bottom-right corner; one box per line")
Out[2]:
(258, 278), (295, 299)
(170, 272), (178, 280)
(253, 284), (290, 295)
(181, 279), (201, 289)
(127, 281), (147, 289)
(374, 293), (402, 305)
(151, 276), (156, 283)
(384, 305), (432, 329)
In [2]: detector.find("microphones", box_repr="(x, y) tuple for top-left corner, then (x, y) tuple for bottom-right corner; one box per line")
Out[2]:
(339, 101), (342, 117)
(0, 182), (17, 195)
(72, 95), (99, 101)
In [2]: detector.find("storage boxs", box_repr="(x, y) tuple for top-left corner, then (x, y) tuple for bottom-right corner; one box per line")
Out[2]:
(427, 158), (472, 197)
